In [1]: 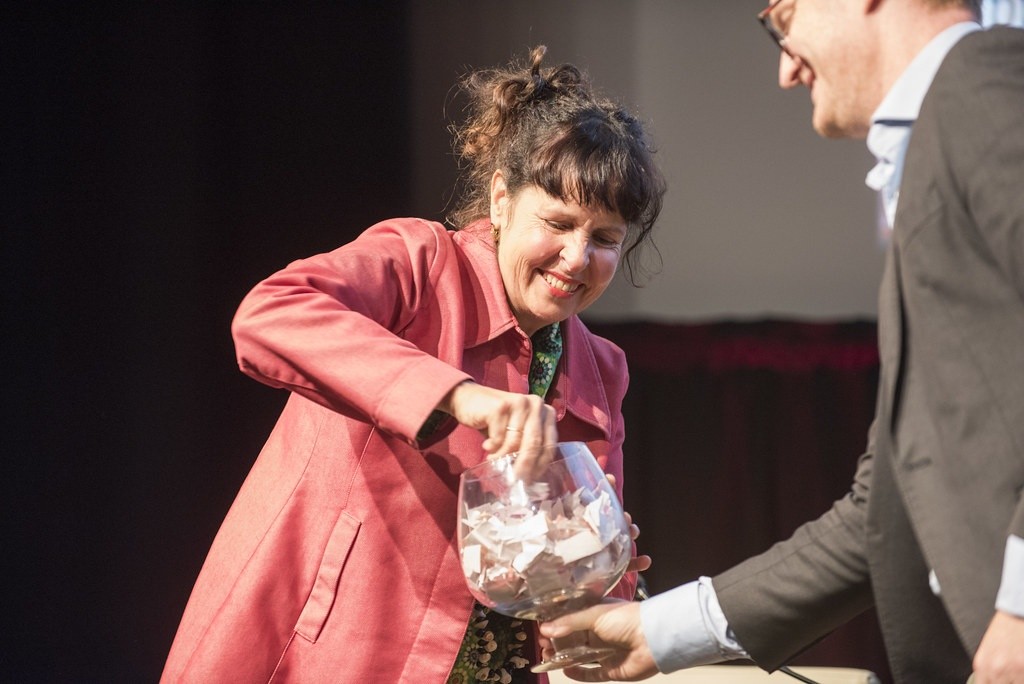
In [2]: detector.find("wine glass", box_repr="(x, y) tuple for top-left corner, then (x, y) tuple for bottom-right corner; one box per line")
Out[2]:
(456, 440), (631, 675)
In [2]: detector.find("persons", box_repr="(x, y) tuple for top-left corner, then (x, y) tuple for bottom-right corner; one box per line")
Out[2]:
(159, 45), (669, 684)
(536, 1), (1024, 684)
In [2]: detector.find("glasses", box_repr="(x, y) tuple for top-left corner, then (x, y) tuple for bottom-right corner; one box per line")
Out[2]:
(756, 0), (780, 47)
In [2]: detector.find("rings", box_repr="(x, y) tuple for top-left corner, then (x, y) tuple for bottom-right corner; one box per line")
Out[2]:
(506, 426), (524, 433)
